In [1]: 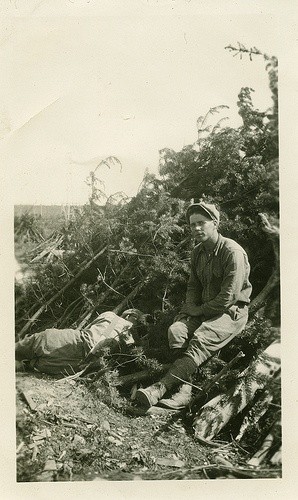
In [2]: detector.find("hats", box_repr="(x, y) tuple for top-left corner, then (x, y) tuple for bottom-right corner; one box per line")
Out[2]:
(121, 309), (144, 319)
(185, 202), (220, 225)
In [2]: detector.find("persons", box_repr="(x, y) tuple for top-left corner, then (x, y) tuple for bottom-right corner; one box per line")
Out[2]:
(15, 306), (147, 377)
(136, 202), (250, 410)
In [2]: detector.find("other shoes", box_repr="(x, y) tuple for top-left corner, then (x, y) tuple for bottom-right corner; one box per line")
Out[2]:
(159, 385), (192, 409)
(136, 382), (166, 406)
